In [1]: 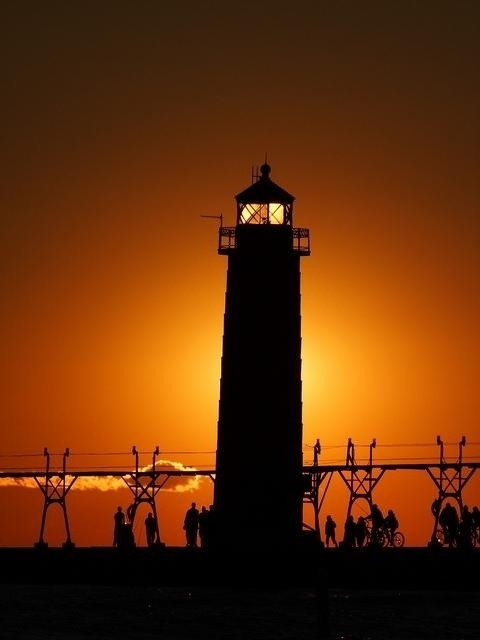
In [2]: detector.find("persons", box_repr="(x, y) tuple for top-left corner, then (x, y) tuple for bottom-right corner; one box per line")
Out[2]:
(198, 505), (211, 548)
(343, 515), (357, 547)
(182, 502), (200, 547)
(324, 514), (338, 548)
(355, 516), (371, 547)
(366, 504), (385, 546)
(111, 506), (126, 547)
(383, 509), (399, 546)
(144, 512), (157, 547)
(438, 501), (480, 545)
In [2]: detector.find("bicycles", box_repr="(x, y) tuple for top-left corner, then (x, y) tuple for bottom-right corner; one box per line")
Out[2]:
(361, 517), (404, 547)
